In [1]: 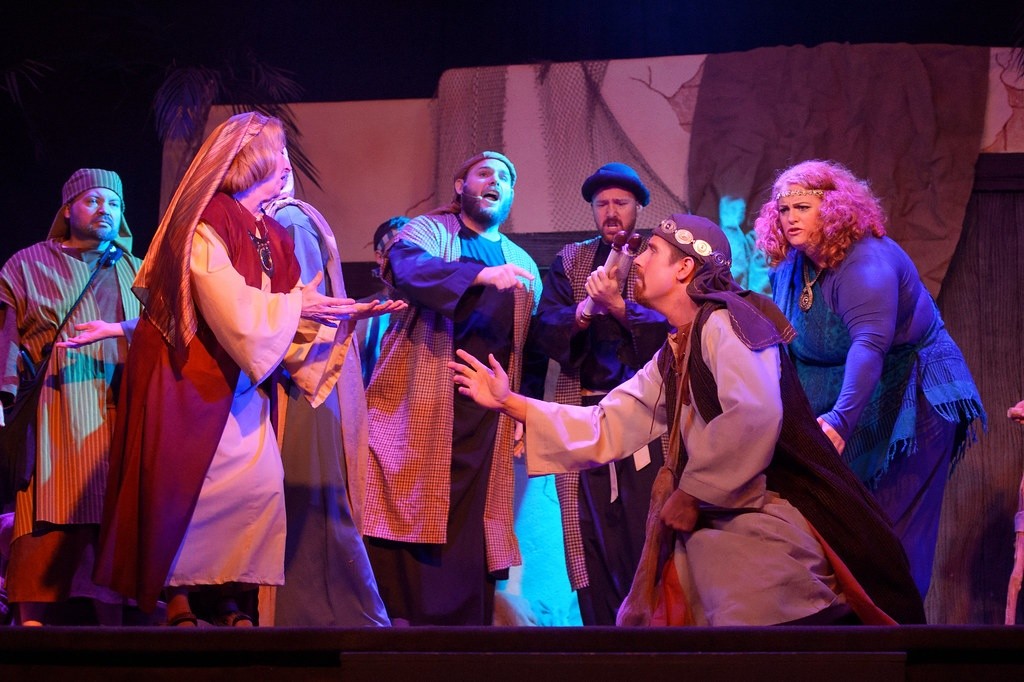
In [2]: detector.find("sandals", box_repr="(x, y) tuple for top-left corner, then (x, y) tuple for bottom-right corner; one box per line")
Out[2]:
(160, 612), (197, 628)
(232, 615), (253, 627)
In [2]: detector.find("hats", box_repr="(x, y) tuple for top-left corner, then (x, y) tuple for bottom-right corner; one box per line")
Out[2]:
(454, 150), (517, 207)
(582, 162), (651, 208)
(374, 216), (412, 250)
(652, 212), (798, 350)
(47, 168), (134, 256)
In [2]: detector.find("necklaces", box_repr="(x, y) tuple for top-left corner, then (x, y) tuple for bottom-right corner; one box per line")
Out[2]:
(232, 197), (279, 272)
(795, 256), (833, 311)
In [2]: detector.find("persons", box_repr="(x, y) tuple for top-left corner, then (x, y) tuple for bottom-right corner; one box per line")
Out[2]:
(356, 148), (547, 627)
(134, 109), (413, 631)
(750, 161), (990, 614)
(231, 142), (399, 626)
(535, 160), (682, 624)
(339, 215), (415, 392)
(445, 212), (928, 626)
(0, 168), (151, 630)
